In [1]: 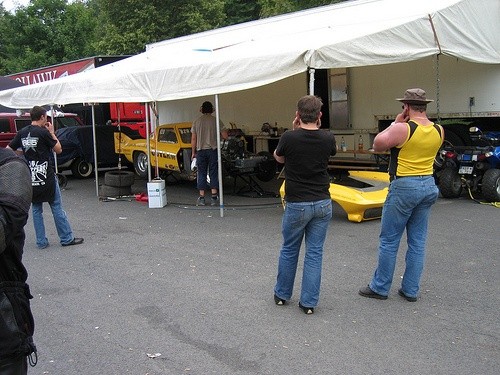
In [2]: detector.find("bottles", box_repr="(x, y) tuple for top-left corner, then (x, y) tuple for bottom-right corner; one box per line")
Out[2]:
(358, 135), (363, 153)
(274, 122), (278, 137)
(340, 137), (346, 153)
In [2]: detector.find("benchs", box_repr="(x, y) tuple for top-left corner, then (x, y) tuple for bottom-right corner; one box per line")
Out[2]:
(328, 158), (390, 171)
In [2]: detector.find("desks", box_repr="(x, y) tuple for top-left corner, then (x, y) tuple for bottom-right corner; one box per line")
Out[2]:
(336, 148), (391, 167)
(236, 172), (280, 196)
(253, 135), (281, 154)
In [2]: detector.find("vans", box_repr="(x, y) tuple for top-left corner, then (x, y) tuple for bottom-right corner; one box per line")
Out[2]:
(0, 112), (85, 149)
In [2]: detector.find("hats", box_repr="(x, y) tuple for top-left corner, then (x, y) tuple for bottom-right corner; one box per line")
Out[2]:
(396, 88), (433, 104)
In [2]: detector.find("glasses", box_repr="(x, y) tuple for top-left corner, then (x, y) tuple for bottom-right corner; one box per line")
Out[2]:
(402, 103), (406, 109)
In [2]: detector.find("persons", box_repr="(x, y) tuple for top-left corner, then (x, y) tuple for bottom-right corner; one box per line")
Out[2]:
(273, 96), (336, 314)
(7, 106), (84, 248)
(0, 148), (37, 375)
(192, 102), (227, 206)
(359, 89), (445, 301)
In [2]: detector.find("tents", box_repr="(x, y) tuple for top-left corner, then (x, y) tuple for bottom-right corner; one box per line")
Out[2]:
(0, 0), (500, 216)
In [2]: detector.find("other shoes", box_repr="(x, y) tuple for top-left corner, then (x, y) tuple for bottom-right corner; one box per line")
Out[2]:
(399, 288), (417, 302)
(275, 295), (285, 305)
(63, 238), (82, 245)
(360, 286), (387, 300)
(299, 302), (313, 314)
(211, 197), (219, 205)
(198, 197), (205, 206)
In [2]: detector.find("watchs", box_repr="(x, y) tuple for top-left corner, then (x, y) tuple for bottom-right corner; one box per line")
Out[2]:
(391, 121), (395, 125)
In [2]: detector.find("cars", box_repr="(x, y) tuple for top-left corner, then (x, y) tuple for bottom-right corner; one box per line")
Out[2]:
(114, 122), (277, 182)
(17, 122), (142, 180)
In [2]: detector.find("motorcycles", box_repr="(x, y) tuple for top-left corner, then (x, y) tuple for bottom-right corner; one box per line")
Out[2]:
(437, 126), (500, 203)
